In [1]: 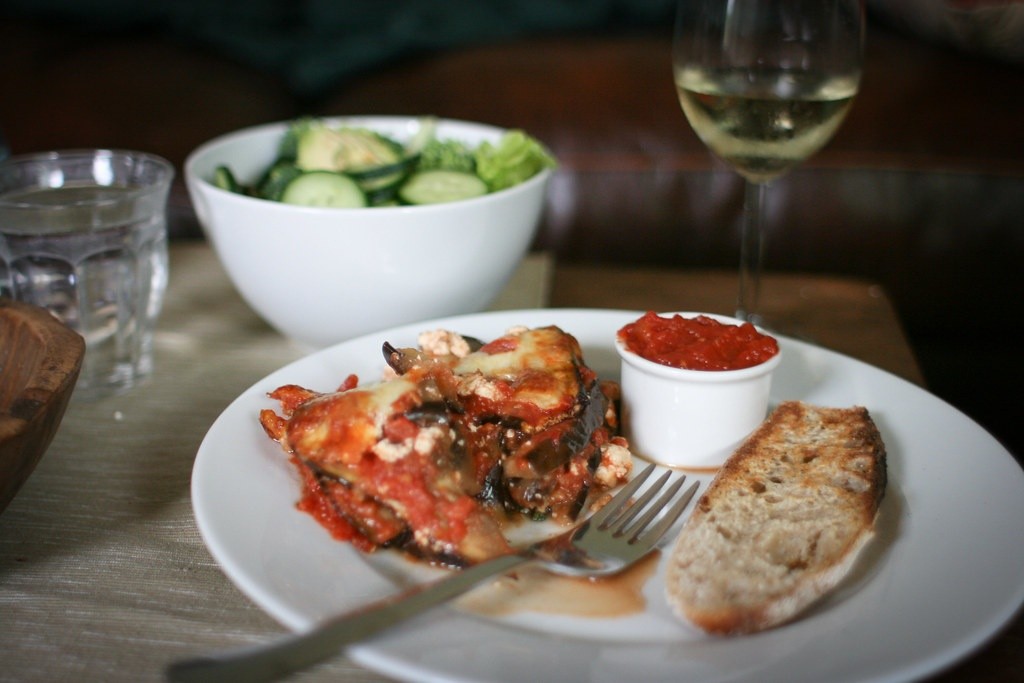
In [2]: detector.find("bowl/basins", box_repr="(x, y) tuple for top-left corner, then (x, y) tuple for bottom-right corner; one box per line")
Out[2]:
(0, 297), (85, 516)
(187, 114), (559, 354)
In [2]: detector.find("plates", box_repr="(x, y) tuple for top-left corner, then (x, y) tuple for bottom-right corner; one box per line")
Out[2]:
(192, 308), (1024, 683)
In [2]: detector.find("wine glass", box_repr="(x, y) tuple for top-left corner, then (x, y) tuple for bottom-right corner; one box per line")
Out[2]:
(672, 0), (868, 326)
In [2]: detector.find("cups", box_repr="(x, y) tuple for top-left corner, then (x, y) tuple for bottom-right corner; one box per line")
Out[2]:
(616, 312), (782, 471)
(0, 147), (176, 401)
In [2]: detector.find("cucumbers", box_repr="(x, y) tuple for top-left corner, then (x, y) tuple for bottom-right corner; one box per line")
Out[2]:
(216, 116), (495, 218)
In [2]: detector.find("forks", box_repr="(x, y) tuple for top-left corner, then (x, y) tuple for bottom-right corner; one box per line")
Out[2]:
(161, 464), (702, 683)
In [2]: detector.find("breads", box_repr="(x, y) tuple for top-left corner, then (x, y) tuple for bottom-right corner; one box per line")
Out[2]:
(665, 401), (887, 636)
